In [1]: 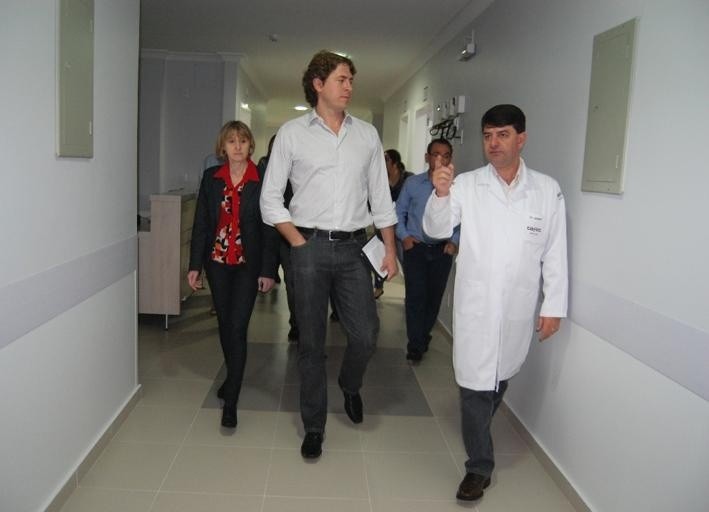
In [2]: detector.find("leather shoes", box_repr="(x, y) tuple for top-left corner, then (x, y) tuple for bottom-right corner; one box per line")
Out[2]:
(457, 472), (491, 501)
(300, 432), (324, 458)
(337, 377), (363, 424)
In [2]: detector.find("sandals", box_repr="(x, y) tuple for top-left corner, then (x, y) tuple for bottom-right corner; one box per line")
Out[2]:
(373, 290), (384, 300)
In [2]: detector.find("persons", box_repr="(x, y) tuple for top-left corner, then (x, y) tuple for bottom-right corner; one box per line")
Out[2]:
(188, 121), (278, 427)
(422, 104), (568, 501)
(258, 52), (398, 458)
(394, 138), (460, 361)
(375, 148), (414, 298)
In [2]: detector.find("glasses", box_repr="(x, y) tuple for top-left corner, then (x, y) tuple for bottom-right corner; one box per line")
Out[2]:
(428, 151), (451, 159)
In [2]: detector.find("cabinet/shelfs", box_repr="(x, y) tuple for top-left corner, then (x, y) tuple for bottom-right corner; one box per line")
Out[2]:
(138, 190), (204, 331)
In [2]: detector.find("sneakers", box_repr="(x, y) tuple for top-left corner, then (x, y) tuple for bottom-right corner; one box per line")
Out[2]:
(216, 377), (237, 428)
(405, 333), (433, 361)
(287, 310), (340, 342)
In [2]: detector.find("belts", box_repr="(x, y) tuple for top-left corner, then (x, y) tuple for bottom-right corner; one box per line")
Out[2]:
(295, 226), (366, 241)
